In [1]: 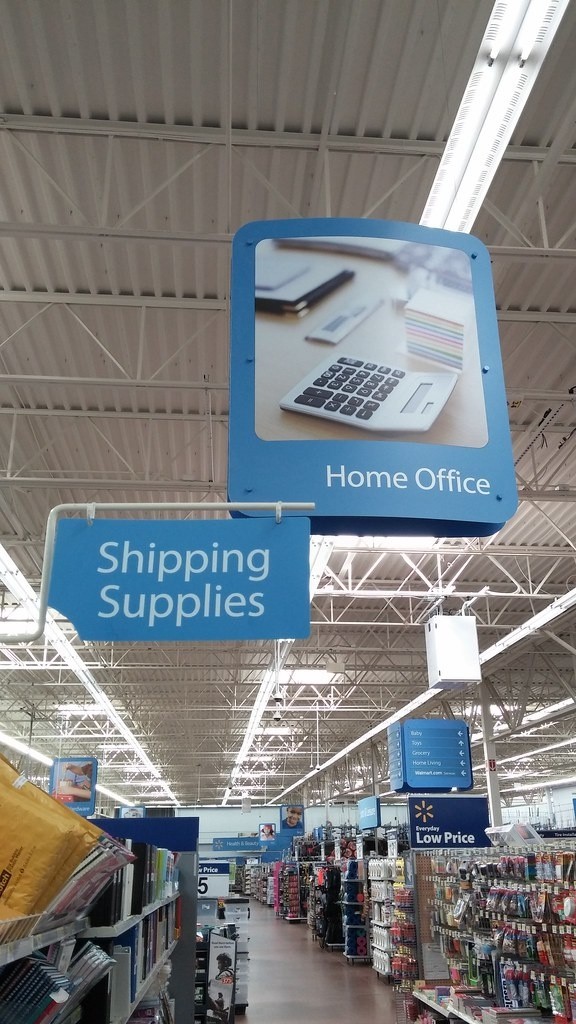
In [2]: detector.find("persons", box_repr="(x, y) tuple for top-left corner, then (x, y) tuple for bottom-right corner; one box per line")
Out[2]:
(216, 952), (236, 979)
(214, 992), (228, 1013)
(260, 825), (276, 839)
(283, 806), (303, 827)
(55, 760), (93, 802)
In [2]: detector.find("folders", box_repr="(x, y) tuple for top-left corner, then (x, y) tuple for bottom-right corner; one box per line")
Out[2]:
(112, 912), (154, 1003)
(91, 836), (158, 927)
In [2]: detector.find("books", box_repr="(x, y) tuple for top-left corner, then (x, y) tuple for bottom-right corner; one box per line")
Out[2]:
(0, 832), (182, 1024)
(403, 287), (472, 375)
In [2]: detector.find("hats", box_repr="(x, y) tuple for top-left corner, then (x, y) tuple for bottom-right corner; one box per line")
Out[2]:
(261, 825), (274, 834)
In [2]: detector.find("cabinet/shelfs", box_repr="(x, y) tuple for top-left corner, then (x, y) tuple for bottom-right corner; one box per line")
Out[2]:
(0, 816), (253, 1024)
(237, 827), (576, 1024)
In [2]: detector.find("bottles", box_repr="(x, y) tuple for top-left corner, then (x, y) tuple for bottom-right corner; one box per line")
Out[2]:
(373, 902), (392, 973)
(369, 858), (394, 899)
